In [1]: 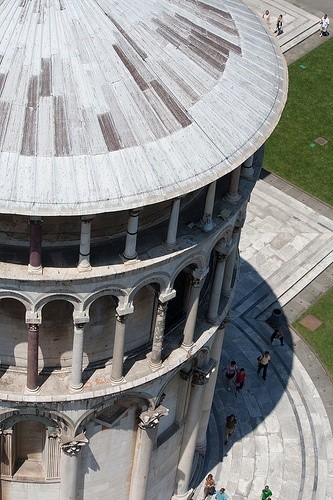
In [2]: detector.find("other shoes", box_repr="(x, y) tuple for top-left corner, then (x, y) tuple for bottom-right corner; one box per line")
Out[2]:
(262, 376), (266, 380)
(229, 433), (231, 436)
(224, 440), (228, 445)
(228, 388), (230, 391)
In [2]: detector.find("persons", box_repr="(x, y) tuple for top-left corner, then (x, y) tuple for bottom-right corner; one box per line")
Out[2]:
(232, 367), (247, 397)
(201, 473), (216, 500)
(223, 414), (238, 445)
(261, 485), (272, 500)
(257, 350), (272, 381)
(221, 360), (239, 393)
(269, 328), (286, 347)
(214, 488), (227, 500)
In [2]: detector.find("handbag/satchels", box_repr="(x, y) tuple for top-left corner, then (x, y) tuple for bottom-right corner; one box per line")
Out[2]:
(257, 355), (264, 360)
(226, 371), (235, 379)
